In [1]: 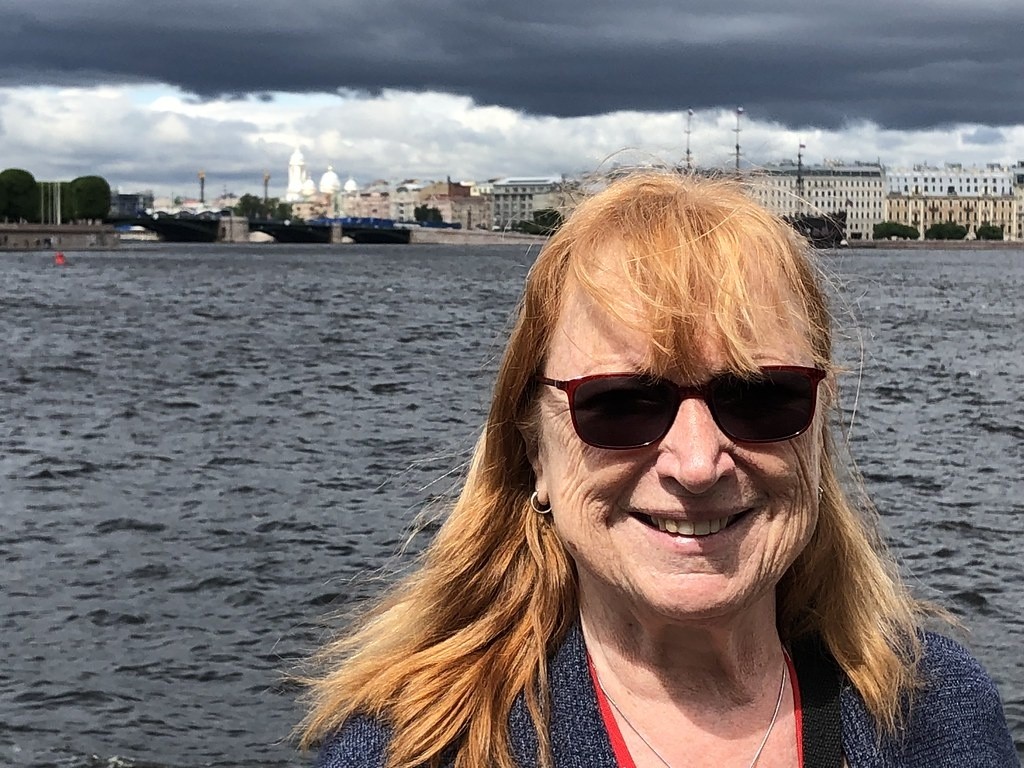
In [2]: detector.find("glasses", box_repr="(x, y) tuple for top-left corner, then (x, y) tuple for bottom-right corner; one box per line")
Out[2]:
(532, 366), (827, 450)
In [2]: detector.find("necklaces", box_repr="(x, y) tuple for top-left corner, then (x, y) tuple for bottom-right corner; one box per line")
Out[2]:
(591, 662), (787, 768)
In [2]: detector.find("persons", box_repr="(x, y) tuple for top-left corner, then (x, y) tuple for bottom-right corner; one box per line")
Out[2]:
(291, 169), (1024, 768)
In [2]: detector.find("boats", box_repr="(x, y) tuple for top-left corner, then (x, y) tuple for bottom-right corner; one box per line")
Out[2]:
(140, 201), (412, 244)
